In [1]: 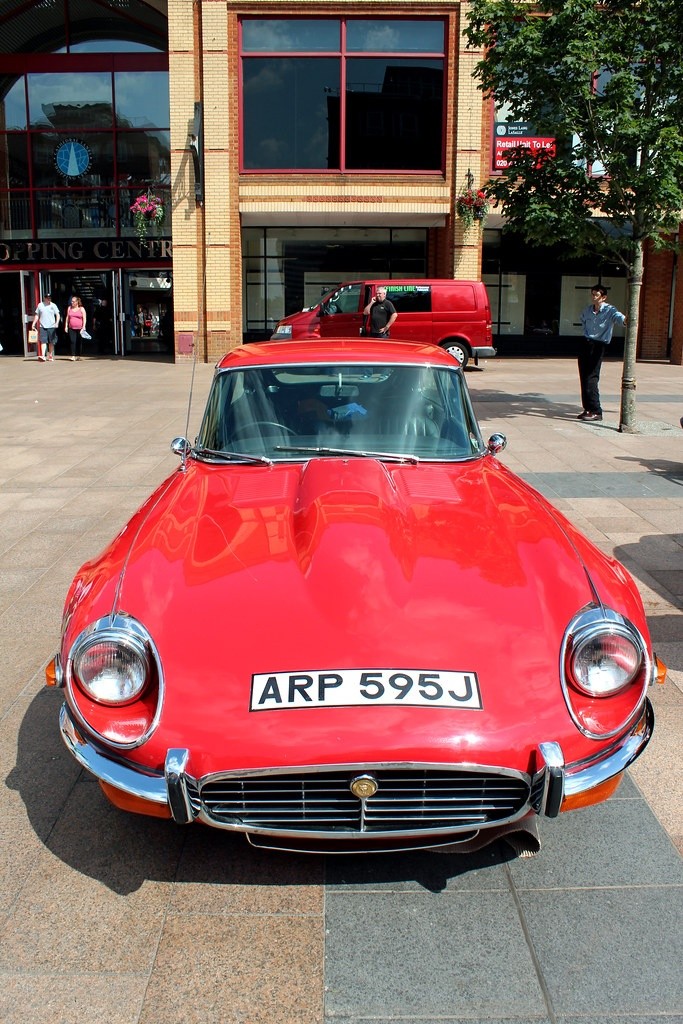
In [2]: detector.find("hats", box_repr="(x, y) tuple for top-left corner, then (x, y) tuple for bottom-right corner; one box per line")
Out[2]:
(44, 293), (52, 299)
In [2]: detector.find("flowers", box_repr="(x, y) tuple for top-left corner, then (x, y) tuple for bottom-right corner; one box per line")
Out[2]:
(455, 188), (491, 237)
(130, 193), (167, 250)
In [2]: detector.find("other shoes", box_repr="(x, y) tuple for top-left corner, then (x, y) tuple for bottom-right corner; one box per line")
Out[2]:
(49, 354), (53, 360)
(77, 356), (81, 361)
(38, 356), (46, 362)
(70, 356), (75, 361)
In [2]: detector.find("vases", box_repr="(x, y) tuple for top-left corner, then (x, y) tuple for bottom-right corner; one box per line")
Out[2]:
(145, 210), (153, 220)
(458, 205), (481, 221)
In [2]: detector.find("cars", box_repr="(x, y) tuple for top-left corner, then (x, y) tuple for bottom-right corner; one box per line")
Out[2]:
(40, 337), (669, 862)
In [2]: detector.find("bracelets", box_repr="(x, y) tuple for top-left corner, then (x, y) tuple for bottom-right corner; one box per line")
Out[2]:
(385, 325), (390, 328)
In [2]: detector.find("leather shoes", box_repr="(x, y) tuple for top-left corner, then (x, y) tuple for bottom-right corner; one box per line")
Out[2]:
(577, 410), (590, 419)
(583, 413), (602, 421)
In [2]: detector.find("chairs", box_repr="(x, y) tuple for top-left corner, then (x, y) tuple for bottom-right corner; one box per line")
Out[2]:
(244, 386), (314, 438)
(369, 384), (442, 437)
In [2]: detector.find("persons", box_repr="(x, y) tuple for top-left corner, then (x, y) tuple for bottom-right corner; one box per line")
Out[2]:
(576, 285), (625, 420)
(92, 299), (174, 358)
(32, 294), (60, 362)
(65, 296), (86, 361)
(363, 287), (397, 339)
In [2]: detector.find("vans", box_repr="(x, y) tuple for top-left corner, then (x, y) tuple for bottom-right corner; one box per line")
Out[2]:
(270, 278), (498, 373)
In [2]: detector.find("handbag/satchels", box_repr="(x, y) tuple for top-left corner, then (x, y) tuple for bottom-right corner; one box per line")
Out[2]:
(80, 328), (92, 339)
(28, 328), (38, 343)
